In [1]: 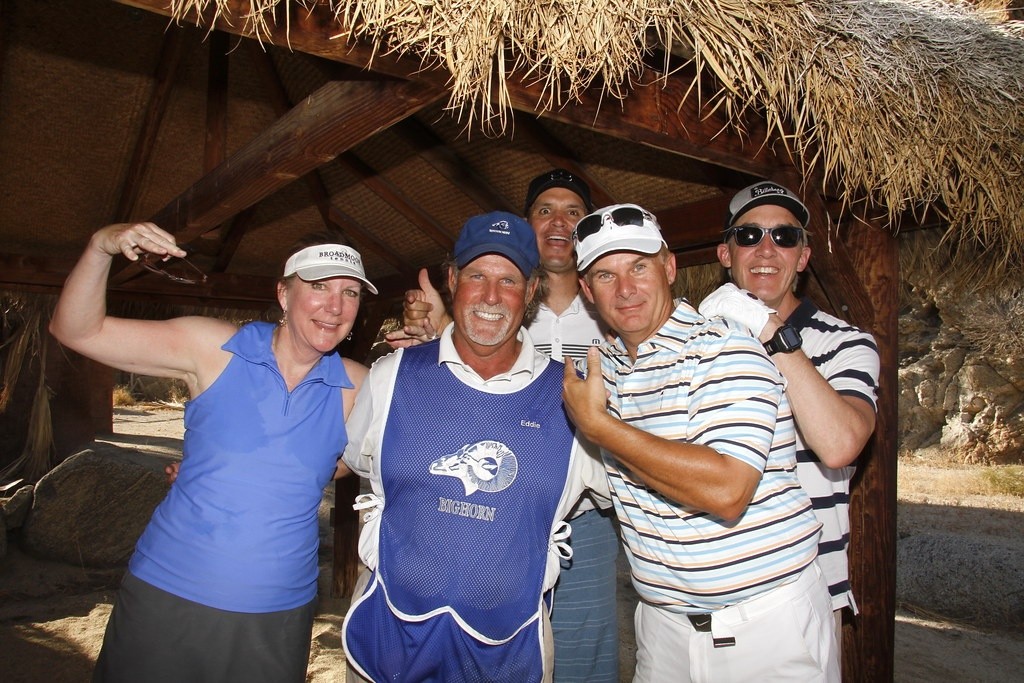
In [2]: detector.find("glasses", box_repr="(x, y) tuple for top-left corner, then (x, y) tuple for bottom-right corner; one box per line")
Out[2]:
(132, 244), (208, 285)
(726, 226), (805, 248)
(571, 207), (661, 242)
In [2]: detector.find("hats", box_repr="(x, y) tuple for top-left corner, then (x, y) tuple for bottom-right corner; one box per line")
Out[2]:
(725, 181), (810, 236)
(572, 204), (669, 271)
(283, 244), (378, 294)
(453, 211), (539, 279)
(524, 169), (592, 217)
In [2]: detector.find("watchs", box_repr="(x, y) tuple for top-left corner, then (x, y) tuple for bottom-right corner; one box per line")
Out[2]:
(762, 324), (802, 357)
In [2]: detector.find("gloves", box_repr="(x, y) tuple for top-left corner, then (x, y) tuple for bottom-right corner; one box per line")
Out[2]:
(698, 282), (779, 339)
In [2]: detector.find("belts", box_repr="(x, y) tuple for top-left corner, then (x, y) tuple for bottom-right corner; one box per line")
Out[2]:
(651, 561), (820, 648)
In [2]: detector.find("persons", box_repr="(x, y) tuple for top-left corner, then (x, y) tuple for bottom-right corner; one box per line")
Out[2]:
(48, 168), (881, 683)
